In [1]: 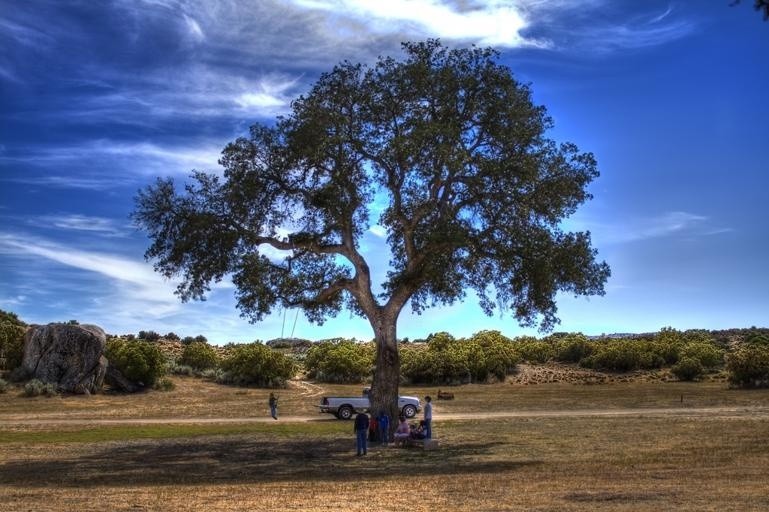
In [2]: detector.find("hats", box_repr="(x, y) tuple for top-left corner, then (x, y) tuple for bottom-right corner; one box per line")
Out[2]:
(356, 407), (368, 414)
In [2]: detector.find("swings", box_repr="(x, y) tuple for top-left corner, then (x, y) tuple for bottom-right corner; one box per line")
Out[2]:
(270, 307), (300, 407)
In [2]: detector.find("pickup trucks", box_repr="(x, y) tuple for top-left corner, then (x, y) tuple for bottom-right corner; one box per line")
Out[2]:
(313, 387), (423, 420)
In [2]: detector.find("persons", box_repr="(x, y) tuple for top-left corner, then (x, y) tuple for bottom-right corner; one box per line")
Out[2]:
(268, 392), (278, 421)
(353, 407), (369, 456)
(369, 395), (432, 446)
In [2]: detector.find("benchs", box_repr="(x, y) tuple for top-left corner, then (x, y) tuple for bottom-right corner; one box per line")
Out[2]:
(396, 436), (439, 451)
